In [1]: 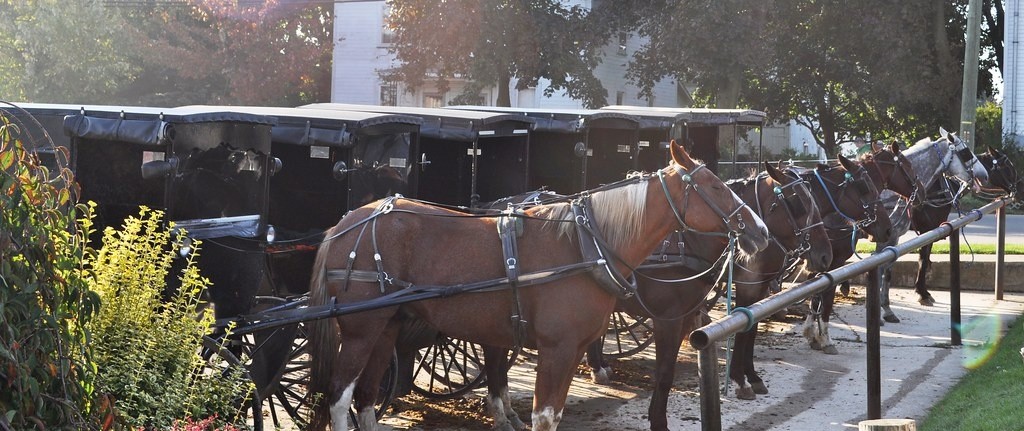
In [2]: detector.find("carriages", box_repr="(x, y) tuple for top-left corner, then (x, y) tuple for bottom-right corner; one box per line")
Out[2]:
(2, 102), (994, 431)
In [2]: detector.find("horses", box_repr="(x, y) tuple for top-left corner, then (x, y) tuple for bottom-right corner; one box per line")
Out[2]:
(306, 141), (768, 431)
(473, 126), (1024, 431)
